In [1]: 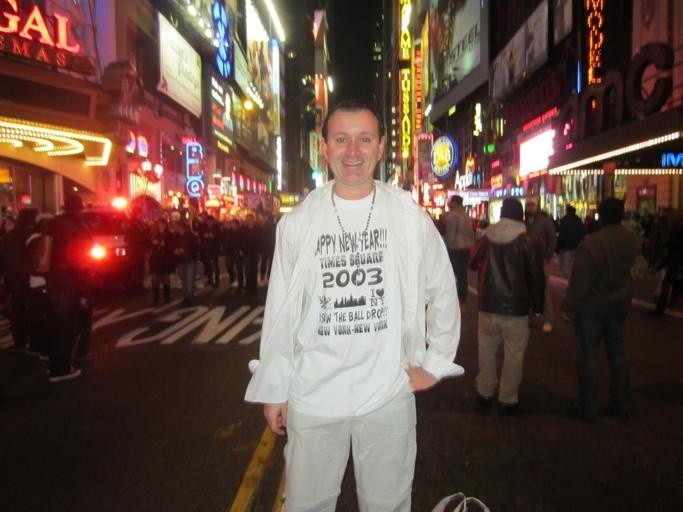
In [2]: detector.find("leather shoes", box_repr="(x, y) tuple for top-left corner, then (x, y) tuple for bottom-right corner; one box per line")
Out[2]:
(600, 404), (630, 421)
(566, 400), (595, 422)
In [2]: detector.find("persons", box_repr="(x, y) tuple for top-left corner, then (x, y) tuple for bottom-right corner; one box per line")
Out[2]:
(557, 197), (642, 423)
(431, 20), (458, 105)
(429, 194), (682, 321)
(1, 194), (96, 383)
(524, 201), (559, 335)
(125, 195), (283, 306)
(244, 98), (466, 512)
(467, 196), (545, 415)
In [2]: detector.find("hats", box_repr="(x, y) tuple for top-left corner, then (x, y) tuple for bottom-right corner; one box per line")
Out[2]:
(500, 195), (523, 221)
(64, 194), (82, 210)
(595, 195), (625, 223)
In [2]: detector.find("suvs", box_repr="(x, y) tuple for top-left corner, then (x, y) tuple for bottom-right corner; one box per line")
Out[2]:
(55, 207), (148, 300)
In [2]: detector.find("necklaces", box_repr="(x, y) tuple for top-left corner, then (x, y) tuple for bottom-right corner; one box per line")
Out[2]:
(329, 183), (381, 250)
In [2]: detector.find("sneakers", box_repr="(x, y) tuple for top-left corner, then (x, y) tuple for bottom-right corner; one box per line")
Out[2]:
(48, 367), (82, 383)
